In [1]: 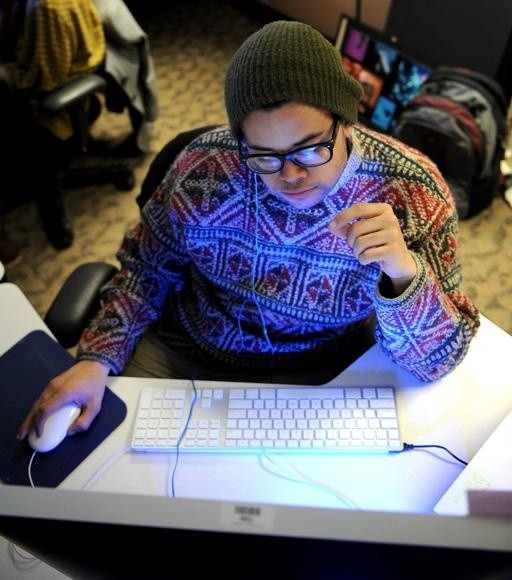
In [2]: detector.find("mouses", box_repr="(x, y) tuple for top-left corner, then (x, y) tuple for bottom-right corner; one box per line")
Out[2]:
(25, 402), (84, 452)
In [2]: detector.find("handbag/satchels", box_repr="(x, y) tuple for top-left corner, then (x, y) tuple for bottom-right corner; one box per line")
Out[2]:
(388, 66), (506, 220)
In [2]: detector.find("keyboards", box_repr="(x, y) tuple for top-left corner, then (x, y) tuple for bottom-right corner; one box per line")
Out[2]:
(129, 384), (405, 455)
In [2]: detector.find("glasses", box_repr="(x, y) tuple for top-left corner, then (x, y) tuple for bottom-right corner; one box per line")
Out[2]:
(233, 116), (339, 174)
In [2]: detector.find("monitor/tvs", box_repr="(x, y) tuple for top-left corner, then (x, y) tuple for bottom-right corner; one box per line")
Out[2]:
(0, 486), (511, 580)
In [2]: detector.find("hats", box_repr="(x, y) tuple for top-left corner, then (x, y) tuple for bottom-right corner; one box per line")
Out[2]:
(223, 21), (363, 141)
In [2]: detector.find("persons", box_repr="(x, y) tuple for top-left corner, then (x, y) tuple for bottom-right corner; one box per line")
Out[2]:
(15, 19), (481, 440)
(0, 0), (106, 140)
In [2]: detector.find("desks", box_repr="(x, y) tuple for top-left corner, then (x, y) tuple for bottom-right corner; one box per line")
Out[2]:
(0, 282), (512, 580)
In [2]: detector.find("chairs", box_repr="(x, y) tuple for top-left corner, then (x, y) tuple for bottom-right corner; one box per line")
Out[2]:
(44, 126), (223, 348)
(35, 73), (136, 249)
